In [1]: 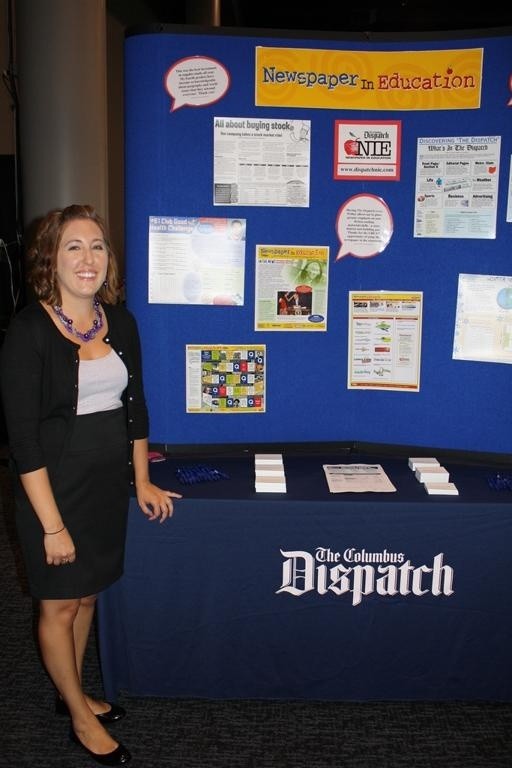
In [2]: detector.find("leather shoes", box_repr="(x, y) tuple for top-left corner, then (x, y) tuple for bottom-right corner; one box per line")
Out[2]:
(92, 698), (127, 724)
(69, 724), (133, 768)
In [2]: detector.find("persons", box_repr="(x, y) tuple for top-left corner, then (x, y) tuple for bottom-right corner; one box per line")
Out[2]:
(300, 260), (321, 286)
(226, 219), (246, 241)
(0, 199), (186, 766)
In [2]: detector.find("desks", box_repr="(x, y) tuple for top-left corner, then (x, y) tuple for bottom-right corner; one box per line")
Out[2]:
(74, 449), (512, 711)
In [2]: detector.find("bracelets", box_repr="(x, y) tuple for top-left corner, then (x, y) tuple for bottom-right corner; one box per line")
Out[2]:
(42, 525), (67, 535)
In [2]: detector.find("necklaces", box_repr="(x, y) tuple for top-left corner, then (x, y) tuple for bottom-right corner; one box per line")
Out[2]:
(49, 298), (104, 341)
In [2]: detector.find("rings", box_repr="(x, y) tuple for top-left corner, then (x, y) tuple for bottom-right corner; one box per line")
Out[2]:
(60, 558), (70, 565)
